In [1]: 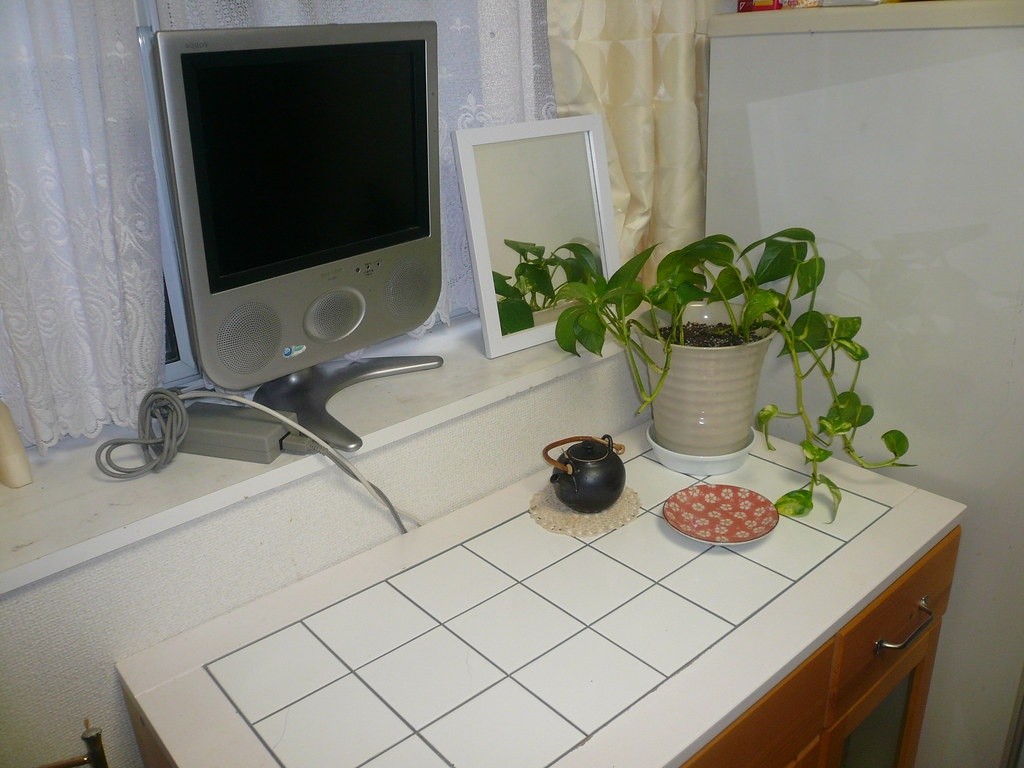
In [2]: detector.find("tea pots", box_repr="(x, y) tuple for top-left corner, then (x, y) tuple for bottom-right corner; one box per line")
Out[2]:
(542, 433), (626, 514)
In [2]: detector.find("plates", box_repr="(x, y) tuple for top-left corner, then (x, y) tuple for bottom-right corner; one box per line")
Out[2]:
(663, 484), (780, 546)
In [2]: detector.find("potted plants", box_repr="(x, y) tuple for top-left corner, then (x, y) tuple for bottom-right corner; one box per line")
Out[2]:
(499, 223), (919, 527)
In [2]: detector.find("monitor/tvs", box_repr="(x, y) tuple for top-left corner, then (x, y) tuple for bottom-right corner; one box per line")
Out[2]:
(154, 21), (444, 453)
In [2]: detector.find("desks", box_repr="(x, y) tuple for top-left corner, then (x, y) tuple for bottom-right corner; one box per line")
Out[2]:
(112, 412), (971, 767)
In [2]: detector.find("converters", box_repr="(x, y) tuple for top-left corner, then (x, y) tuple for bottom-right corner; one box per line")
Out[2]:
(161, 401), (298, 465)
